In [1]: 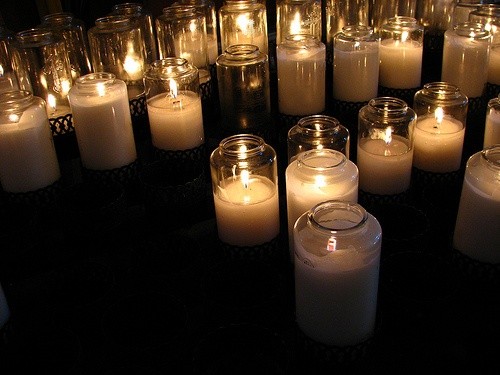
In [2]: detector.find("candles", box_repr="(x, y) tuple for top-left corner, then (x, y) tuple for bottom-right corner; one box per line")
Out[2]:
(0, 0), (500, 346)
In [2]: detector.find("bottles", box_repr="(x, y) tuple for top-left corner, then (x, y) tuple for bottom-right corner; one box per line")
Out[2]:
(293, 200), (382, 345)
(484, 94), (500, 149)
(68, 73), (136, 171)
(276, 0), (500, 116)
(143, 1), (271, 150)
(285, 149), (359, 264)
(0, 13), (91, 194)
(87, 3), (156, 100)
(209, 133), (279, 247)
(287, 115), (350, 161)
(453, 144), (500, 264)
(413, 82), (468, 173)
(357, 96), (417, 195)
